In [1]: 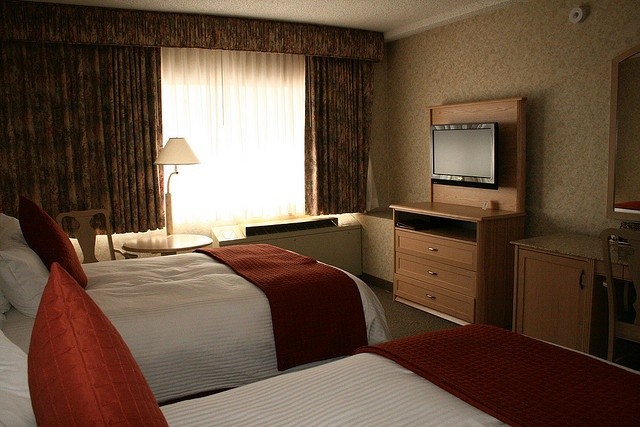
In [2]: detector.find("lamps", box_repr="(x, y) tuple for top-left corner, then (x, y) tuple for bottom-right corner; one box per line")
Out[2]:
(153, 135), (200, 233)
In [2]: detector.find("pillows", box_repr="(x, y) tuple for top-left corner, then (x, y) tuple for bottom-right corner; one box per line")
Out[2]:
(1, 292), (12, 313)
(29, 262), (170, 427)
(0, 245), (50, 318)
(1, 215), (27, 244)
(0, 330), (39, 427)
(17, 197), (89, 289)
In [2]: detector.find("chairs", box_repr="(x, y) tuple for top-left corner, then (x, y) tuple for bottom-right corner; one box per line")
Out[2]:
(601, 228), (640, 372)
(49, 207), (138, 266)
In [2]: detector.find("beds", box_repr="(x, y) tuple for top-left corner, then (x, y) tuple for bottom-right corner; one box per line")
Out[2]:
(1, 322), (640, 427)
(0, 243), (397, 426)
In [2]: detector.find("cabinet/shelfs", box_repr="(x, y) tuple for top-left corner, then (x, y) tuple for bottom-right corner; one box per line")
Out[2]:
(356, 207), (395, 286)
(511, 234), (640, 355)
(391, 96), (529, 328)
(211, 222), (361, 276)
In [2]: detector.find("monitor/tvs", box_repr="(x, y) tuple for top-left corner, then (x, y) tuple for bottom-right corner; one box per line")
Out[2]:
(429, 122), (499, 191)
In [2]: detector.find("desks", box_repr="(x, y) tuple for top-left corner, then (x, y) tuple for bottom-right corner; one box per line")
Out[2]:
(125, 235), (215, 255)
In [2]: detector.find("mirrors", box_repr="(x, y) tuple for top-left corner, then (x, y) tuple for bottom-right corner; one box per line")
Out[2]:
(606, 46), (640, 223)
